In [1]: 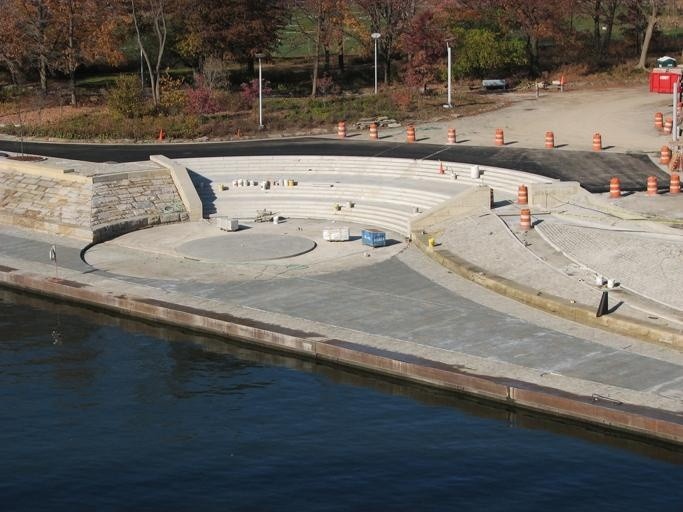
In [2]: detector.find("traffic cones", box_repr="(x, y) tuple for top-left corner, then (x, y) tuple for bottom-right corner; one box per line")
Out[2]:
(439, 163), (445, 174)
(560, 76), (565, 86)
(158, 129), (164, 140)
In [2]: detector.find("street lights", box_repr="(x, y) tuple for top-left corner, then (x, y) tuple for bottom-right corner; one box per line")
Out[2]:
(255, 53), (265, 129)
(443, 39), (456, 108)
(371, 33), (381, 94)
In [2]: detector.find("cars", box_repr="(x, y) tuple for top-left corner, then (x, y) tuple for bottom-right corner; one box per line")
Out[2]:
(483, 74), (508, 89)
